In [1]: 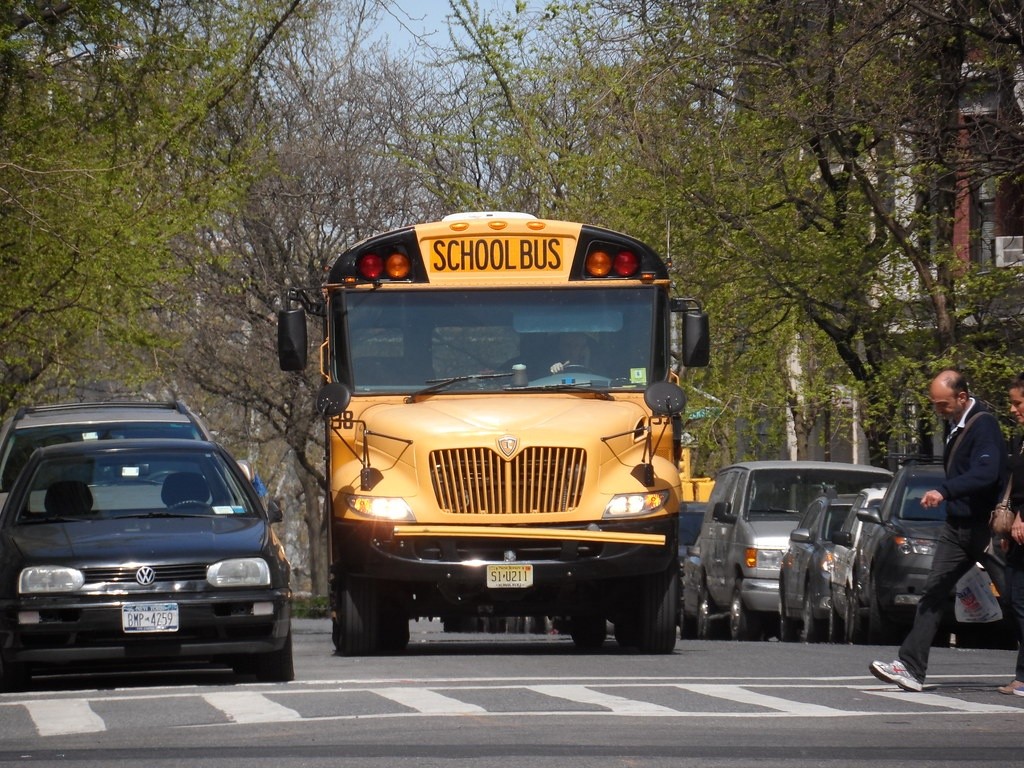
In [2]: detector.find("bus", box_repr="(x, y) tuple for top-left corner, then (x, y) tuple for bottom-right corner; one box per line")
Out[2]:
(276, 208), (711, 658)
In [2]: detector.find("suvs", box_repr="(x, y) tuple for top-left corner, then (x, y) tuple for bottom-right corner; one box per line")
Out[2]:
(869, 456), (948, 640)
(0, 392), (214, 516)
(828, 480), (887, 645)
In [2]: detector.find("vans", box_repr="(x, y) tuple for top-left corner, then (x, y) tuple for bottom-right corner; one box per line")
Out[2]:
(699, 458), (894, 640)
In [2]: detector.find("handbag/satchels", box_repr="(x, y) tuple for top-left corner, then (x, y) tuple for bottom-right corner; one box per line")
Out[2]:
(984, 502), (1014, 536)
(954, 565), (1003, 623)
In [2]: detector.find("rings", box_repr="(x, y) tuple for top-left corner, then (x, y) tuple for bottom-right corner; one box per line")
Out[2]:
(1017, 531), (1020, 533)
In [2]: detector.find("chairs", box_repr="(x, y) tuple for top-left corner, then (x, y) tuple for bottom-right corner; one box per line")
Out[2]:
(44, 480), (94, 519)
(352, 356), (437, 385)
(161, 473), (211, 511)
(908, 498), (942, 519)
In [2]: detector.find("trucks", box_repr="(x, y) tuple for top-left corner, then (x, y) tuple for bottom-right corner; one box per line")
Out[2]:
(679, 446), (717, 501)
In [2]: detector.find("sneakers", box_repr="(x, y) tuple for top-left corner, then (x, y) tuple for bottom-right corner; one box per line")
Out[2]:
(868, 660), (922, 692)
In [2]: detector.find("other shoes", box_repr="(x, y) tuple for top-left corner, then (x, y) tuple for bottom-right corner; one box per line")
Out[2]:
(1013, 686), (1024, 696)
(998, 681), (1024, 694)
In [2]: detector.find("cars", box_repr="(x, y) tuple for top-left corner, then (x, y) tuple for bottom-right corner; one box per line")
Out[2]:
(777, 493), (857, 642)
(679, 501), (708, 574)
(0, 440), (294, 681)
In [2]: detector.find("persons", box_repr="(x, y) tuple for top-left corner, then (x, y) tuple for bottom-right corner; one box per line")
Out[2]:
(866, 370), (1009, 693)
(543, 330), (603, 374)
(993, 370), (1024, 698)
